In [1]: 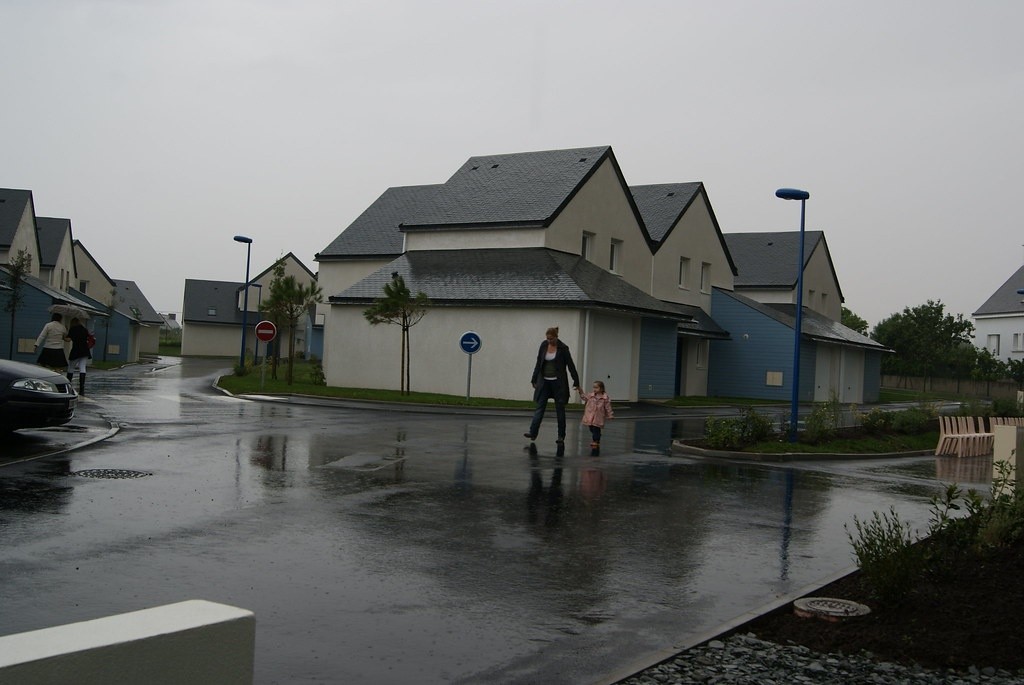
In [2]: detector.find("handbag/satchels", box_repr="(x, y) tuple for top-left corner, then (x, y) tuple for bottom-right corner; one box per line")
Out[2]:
(85, 327), (96, 348)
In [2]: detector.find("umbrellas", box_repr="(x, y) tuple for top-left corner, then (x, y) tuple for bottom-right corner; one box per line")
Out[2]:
(46, 303), (90, 321)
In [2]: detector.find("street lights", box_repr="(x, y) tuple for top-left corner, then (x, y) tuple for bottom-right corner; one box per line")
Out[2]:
(775, 187), (809, 444)
(234, 234), (253, 367)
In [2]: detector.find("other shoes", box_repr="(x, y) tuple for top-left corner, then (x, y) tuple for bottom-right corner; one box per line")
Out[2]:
(590, 441), (598, 446)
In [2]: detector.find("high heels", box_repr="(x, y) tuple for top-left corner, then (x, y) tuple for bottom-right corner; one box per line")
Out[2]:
(555, 440), (564, 445)
(524, 434), (537, 441)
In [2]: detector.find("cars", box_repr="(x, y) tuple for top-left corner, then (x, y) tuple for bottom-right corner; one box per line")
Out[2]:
(0, 359), (79, 435)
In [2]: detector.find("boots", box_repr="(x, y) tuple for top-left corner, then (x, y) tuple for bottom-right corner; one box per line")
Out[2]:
(67, 373), (73, 382)
(79, 373), (86, 394)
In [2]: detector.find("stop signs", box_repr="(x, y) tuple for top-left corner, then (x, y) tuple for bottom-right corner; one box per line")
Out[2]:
(254, 321), (277, 343)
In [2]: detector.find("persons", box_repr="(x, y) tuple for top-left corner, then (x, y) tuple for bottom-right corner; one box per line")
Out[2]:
(578, 381), (615, 446)
(34, 314), (70, 375)
(524, 326), (584, 444)
(63, 318), (92, 396)
